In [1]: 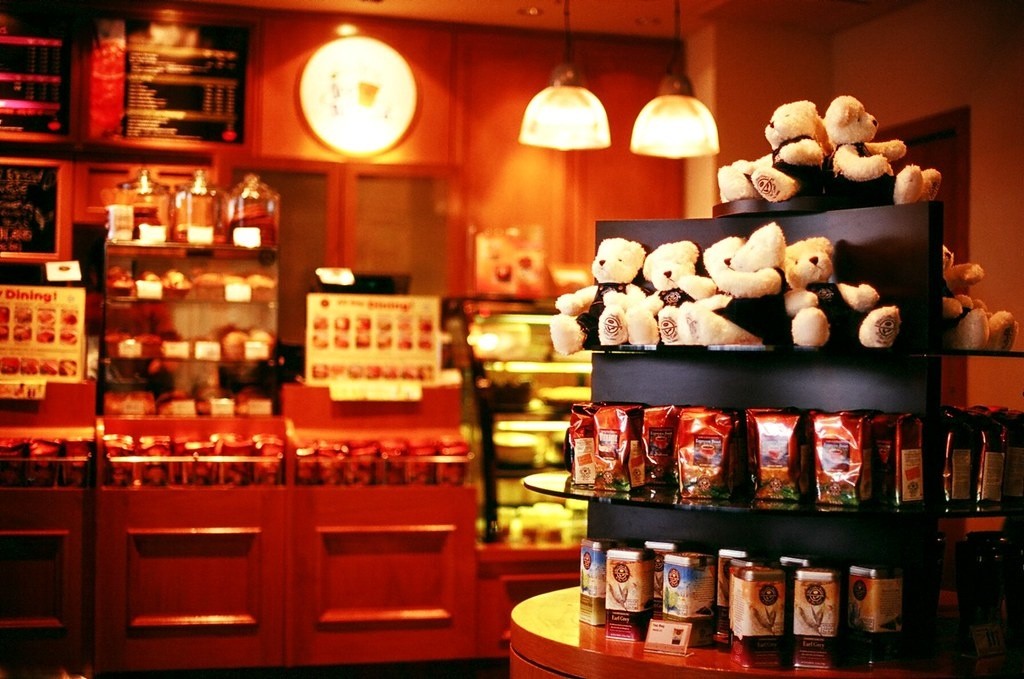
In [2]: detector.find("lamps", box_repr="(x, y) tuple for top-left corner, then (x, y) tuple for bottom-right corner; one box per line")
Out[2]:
(517, 0), (612, 153)
(629, 0), (719, 160)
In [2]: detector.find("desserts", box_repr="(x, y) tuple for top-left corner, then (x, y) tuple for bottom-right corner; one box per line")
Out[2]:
(106, 266), (276, 361)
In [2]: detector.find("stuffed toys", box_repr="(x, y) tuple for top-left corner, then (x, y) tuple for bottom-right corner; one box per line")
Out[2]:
(548, 222), (900, 355)
(942, 244), (1019, 351)
(718, 96), (941, 206)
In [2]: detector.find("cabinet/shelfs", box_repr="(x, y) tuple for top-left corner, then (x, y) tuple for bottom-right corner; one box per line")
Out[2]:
(452, 9), (688, 305)
(508, 203), (1023, 679)
(96, 238), (280, 418)
